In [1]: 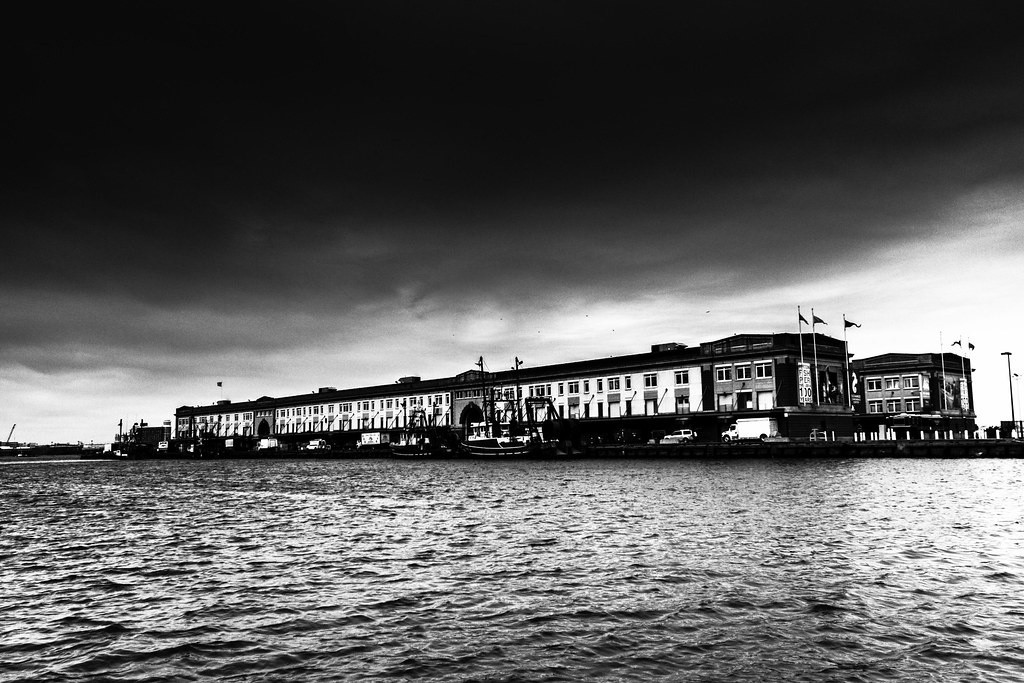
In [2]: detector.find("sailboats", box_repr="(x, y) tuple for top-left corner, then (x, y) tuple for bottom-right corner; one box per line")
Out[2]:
(458, 356), (560, 457)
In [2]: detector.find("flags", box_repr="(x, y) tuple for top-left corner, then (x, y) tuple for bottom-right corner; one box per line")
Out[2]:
(217, 382), (222, 387)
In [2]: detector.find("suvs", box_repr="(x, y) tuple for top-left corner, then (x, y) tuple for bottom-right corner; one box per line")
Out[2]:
(663, 429), (698, 444)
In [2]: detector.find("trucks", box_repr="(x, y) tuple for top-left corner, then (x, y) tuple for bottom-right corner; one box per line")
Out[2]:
(253, 437), (279, 452)
(355, 432), (390, 450)
(722, 417), (779, 443)
(187, 439), (234, 457)
(300, 438), (331, 453)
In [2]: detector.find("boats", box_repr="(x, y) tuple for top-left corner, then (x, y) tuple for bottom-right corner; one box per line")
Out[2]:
(389, 400), (450, 460)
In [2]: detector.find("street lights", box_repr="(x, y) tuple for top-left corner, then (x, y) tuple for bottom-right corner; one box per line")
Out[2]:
(1001, 352), (1016, 422)
